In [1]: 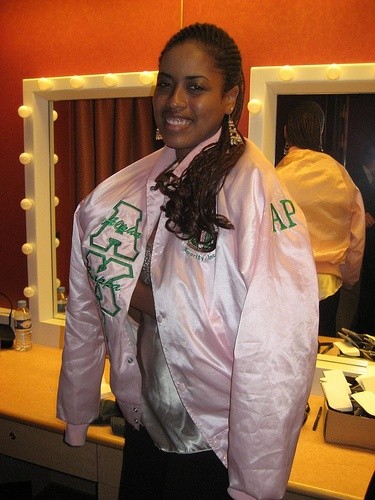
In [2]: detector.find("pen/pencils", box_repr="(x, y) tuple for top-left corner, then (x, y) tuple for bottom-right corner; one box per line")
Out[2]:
(313, 407), (323, 430)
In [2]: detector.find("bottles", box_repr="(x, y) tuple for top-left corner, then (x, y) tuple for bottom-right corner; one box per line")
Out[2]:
(56, 286), (68, 313)
(13, 300), (34, 352)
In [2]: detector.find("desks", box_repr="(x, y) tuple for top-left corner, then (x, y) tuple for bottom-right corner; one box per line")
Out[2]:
(0, 339), (374, 500)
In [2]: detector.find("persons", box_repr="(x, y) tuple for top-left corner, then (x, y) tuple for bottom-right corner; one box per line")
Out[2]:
(52, 19), (319, 500)
(272, 89), (375, 337)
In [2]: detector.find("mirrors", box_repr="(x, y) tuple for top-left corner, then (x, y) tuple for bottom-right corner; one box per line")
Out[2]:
(15, 69), (166, 357)
(247, 60), (375, 390)
